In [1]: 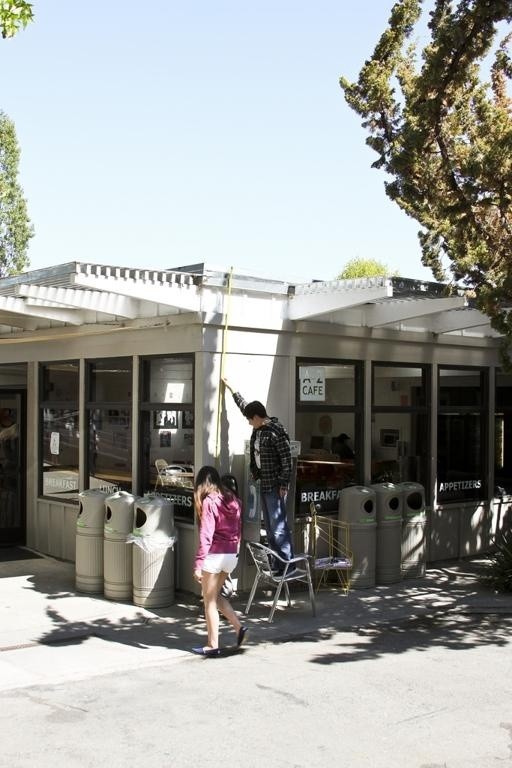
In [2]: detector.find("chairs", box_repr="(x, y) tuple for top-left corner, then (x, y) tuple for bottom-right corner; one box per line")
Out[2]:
(244, 541), (316, 623)
(154, 459), (194, 493)
(310, 502), (352, 595)
(296, 454), (354, 487)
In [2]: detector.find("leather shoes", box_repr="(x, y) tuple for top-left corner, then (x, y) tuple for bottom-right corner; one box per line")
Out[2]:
(237, 626), (249, 646)
(191, 646), (221, 657)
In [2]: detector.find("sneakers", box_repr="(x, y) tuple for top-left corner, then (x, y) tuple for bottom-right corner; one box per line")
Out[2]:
(262, 567), (297, 579)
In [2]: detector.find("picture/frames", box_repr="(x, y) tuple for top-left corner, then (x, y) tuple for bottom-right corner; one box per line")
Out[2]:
(380, 429), (399, 447)
(153, 410), (178, 429)
(182, 411), (193, 429)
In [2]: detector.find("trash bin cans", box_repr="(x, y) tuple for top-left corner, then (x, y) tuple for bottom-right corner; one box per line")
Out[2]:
(338, 481), (429, 589)
(75, 487), (178, 609)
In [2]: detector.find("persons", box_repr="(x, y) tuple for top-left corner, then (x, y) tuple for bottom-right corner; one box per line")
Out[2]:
(331, 433), (353, 462)
(220, 376), (296, 576)
(191, 466), (249, 655)
(0, 423), (22, 539)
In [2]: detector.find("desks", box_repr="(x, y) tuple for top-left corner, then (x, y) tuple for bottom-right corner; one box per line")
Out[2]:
(176, 473), (194, 478)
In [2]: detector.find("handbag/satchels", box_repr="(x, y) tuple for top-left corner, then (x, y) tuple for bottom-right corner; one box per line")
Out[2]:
(221, 579), (233, 600)
